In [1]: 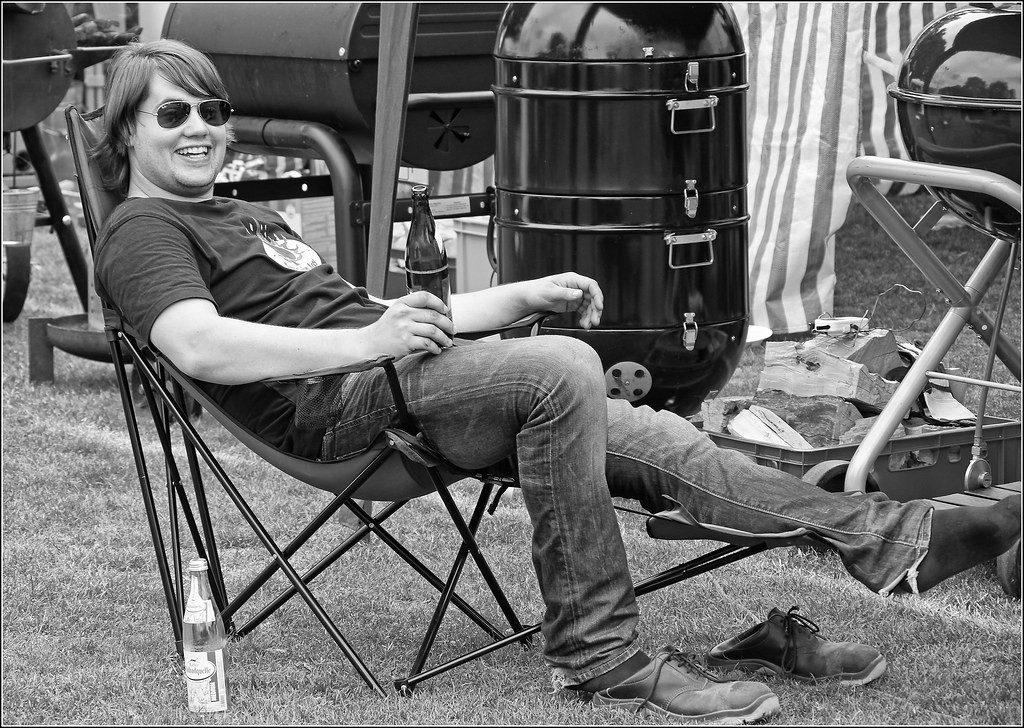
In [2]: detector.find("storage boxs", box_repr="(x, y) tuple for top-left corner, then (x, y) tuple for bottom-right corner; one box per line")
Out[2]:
(684, 411), (1021, 502)
(451, 216), (498, 294)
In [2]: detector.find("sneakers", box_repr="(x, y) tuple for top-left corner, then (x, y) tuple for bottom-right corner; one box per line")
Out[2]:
(705, 604), (888, 686)
(592, 644), (780, 725)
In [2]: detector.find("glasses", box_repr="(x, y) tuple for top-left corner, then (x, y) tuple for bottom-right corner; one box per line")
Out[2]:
(137, 99), (235, 129)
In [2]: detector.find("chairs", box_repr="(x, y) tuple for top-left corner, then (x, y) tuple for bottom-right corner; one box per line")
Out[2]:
(63, 104), (826, 699)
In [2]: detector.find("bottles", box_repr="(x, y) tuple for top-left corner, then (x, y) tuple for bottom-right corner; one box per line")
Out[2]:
(183, 558), (230, 713)
(405, 184), (455, 348)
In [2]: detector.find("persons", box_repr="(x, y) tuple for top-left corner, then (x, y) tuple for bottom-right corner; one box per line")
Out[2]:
(90, 40), (1022, 724)
